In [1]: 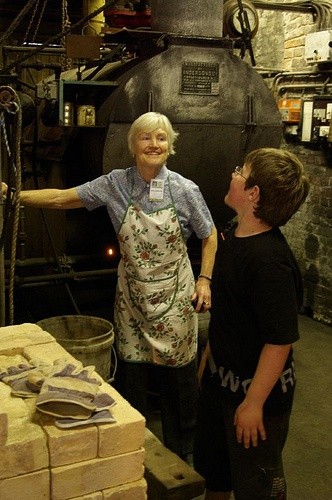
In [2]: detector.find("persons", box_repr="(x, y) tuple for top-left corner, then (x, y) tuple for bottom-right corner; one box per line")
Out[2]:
(196, 147), (311, 500)
(0, 112), (218, 469)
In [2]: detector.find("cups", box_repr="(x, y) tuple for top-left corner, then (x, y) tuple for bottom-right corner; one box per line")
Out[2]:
(77, 105), (95, 127)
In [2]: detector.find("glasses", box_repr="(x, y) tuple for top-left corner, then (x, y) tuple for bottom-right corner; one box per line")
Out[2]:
(234, 166), (248, 180)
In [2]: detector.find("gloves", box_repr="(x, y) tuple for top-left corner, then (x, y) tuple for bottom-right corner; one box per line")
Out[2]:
(0, 357), (117, 429)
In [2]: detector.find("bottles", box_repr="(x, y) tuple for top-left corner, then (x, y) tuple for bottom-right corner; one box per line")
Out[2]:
(64, 102), (74, 125)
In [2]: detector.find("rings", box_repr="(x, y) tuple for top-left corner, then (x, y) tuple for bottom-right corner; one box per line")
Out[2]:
(203, 302), (210, 305)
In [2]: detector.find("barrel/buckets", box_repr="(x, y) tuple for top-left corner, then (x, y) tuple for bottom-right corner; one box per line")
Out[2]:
(37, 315), (117, 385)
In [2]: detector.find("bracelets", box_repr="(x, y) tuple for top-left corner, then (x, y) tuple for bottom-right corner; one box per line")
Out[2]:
(198, 274), (212, 282)
